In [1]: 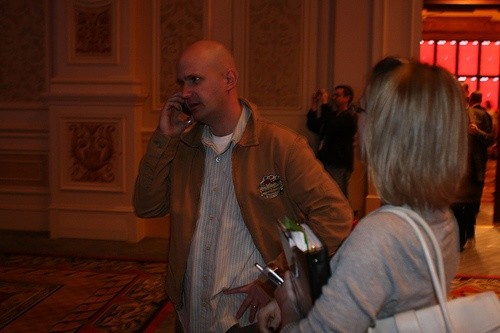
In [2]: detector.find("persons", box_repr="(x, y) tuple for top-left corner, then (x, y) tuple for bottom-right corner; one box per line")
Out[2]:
(449, 85), (500, 252)
(257, 53), (469, 333)
(131, 40), (353, 333)
(305, 84), (358, 199)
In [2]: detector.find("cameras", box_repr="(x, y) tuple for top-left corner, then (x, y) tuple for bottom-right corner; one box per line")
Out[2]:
(316, 90), (322, 97)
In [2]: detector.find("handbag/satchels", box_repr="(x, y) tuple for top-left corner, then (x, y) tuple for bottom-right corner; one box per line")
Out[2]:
(364, 203), (500, 332)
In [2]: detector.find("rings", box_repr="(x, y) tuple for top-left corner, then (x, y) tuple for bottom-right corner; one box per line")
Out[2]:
(249, 305), (258, 313)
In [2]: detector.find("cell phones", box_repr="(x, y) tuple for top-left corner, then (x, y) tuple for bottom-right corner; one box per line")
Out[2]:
(255, 264), (284, 286)
(175, 103), (191, 114)
(263, 263), (284, 279)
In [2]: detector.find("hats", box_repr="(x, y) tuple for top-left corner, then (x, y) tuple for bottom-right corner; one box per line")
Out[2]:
(373, 55), (401, 76)
